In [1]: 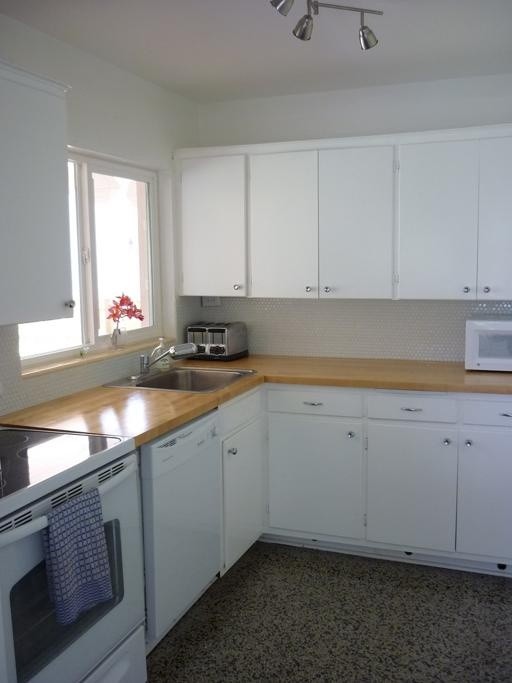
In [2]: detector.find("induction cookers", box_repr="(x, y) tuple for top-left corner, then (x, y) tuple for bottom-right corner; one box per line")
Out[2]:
(0, 424), (135, 523)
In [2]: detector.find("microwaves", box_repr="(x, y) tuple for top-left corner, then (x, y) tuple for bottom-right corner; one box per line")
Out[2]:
(463, 318), (512, 373)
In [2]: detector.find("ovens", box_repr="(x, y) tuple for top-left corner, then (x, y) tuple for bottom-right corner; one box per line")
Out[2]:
(0, 451), (148, 683)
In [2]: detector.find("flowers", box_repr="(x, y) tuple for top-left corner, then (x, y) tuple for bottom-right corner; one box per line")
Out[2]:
(106, 290), (144, 326)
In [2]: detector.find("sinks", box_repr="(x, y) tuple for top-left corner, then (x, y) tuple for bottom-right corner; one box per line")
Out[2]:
(103, 366), (257, 394)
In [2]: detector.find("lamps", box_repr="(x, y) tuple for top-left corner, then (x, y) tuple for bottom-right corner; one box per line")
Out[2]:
(268, 0), (383, 53)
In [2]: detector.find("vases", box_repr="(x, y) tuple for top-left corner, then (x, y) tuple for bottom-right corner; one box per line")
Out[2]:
(110, 328), (122, 346)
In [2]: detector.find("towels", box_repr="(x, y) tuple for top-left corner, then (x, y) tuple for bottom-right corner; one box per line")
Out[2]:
(45, 487), (115, 627)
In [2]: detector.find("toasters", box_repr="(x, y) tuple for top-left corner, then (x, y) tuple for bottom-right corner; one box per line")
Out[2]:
(186, 321), (249, 362)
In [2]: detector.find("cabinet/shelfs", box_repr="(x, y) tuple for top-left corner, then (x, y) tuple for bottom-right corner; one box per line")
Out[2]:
(398, 132), (511, 301)
(177, 145), (248, 298)
(248, 134), (396, 300)
(1, 58), (74, 328)
(365, 388), (512, 580)
(269, 384), (364, 545)
(221, 381), (266, 577)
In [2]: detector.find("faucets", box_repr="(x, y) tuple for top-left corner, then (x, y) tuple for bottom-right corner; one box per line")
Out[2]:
(140, 343), (198, 373)
